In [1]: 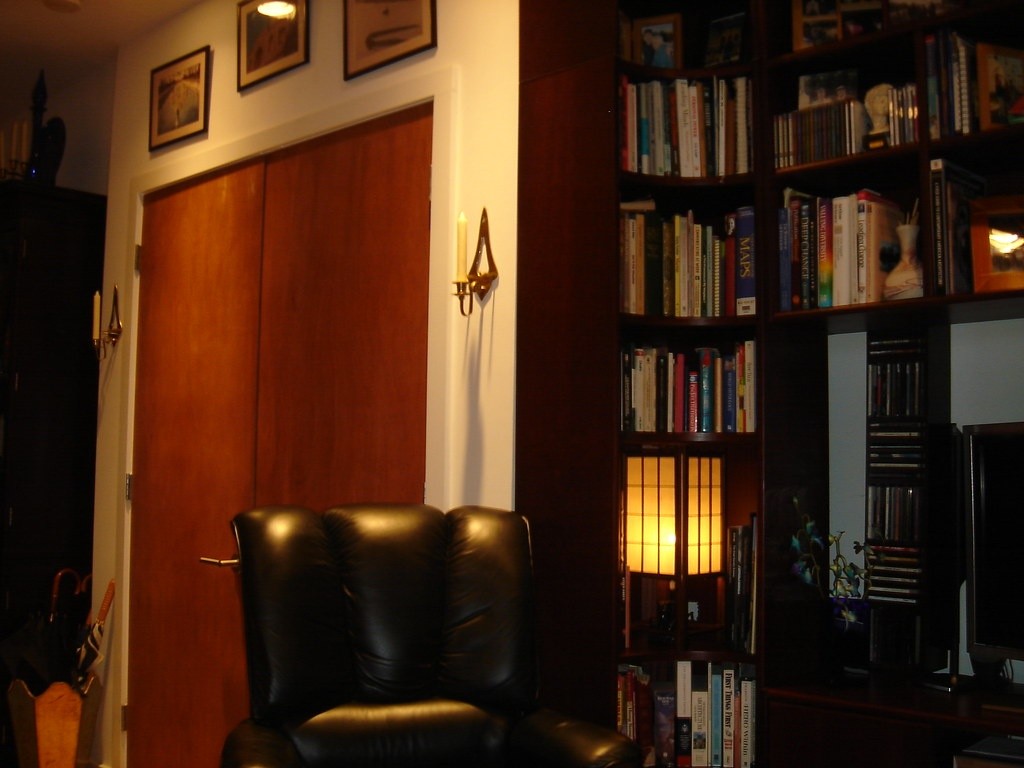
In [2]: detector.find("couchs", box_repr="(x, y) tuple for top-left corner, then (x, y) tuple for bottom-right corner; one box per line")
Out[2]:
(220, 501), (644, 768)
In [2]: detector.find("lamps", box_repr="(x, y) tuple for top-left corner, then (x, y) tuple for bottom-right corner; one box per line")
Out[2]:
(624, 453), (727, 682)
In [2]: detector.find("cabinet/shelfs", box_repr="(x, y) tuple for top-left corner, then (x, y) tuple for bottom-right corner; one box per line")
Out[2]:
(516, 0), (1024, 767)
(0, 68), (107, 768)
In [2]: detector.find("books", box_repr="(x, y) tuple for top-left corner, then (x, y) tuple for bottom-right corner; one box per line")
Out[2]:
(777, 158), (970, 314)
(619, 200), (759, 318)
(865, 545), (931, 604)
(622, 340), (756, 432)
(617, 72), (757, 178)
(615, 659), (757, 768)
(772, 101), (871, 168)
(866, 485), (920, 543)
(867, 423), (925, 477)
(864, 338), (923, 418)
(887, 31), (1024, 147)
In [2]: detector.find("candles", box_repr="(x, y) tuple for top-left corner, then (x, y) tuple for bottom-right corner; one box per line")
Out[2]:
(451, 210), (471, 283)
(92, 290), (101, 338)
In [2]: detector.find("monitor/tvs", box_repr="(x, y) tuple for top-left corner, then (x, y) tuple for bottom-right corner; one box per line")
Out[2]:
(963, 420), (1024, 661)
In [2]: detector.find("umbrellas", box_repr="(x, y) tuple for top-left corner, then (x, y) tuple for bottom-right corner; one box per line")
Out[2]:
(41, 569), (114, 694)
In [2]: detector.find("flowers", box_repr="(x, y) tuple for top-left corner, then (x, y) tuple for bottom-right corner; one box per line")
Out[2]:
(790, 500), (885, 632)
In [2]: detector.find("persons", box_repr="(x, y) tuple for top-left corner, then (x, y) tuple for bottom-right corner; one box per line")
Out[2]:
(864, 82), (894, 130)
(804, 73), (853, 103)
(642, 28), (674, 69)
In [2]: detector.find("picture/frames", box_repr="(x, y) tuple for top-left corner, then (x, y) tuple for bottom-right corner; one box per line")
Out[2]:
(148, 45), (210, 150)
(969, 194), (1024, 292)
(236, 0), (309, 92)
(618, 11), (683, 71)
(795, 67), (862, 111)
(343, 0), (437, 81)
(704, 11), (745, 69)
(792, 1), (843, 51)
